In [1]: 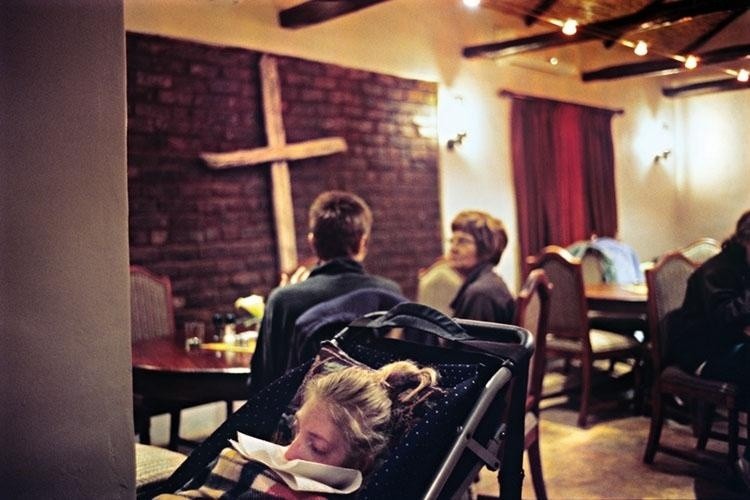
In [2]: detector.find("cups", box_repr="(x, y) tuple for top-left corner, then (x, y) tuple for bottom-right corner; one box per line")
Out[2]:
(183, 321), (206, 357)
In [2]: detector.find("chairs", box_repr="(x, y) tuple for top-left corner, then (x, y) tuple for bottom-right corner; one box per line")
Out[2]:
(129, 235), (750, 499)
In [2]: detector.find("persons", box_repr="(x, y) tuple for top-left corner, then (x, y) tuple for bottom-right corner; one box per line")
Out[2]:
(151, 359), (439, 500)
(448, 210), (515, 324)
(245, 189), (406, 400)
(681, 210), (750, 488)
